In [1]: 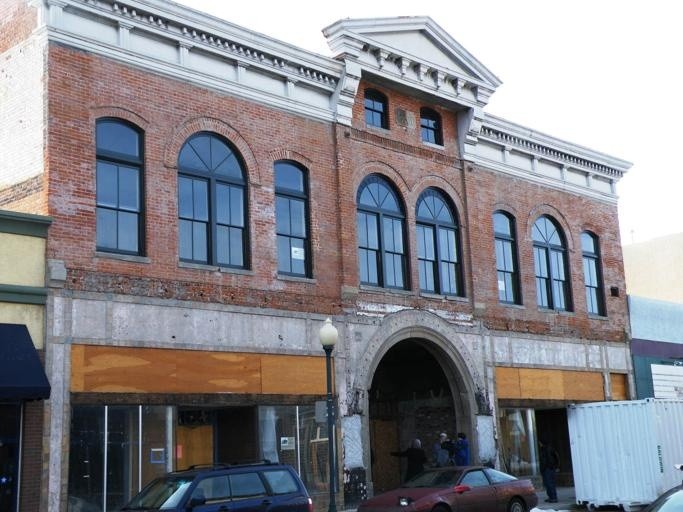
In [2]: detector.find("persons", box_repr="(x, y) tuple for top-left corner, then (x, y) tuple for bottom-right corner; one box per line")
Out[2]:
(537, 438), (560, 504)
(388, 430), (471, 484)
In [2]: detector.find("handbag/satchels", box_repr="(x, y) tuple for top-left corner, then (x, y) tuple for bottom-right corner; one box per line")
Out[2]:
(551, 448), (560, 469)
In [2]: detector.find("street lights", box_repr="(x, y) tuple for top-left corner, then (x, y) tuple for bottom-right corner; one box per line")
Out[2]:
(319, 312), (343, 511)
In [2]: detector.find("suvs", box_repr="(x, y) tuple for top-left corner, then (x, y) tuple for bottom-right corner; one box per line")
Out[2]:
(114, 458), (313, 511)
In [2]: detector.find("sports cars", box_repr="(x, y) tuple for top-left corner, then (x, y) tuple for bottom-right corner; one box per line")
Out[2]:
(352, 464), (541, 512)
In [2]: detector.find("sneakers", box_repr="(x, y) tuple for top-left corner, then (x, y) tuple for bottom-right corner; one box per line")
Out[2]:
(546, 498), (557, 502)
